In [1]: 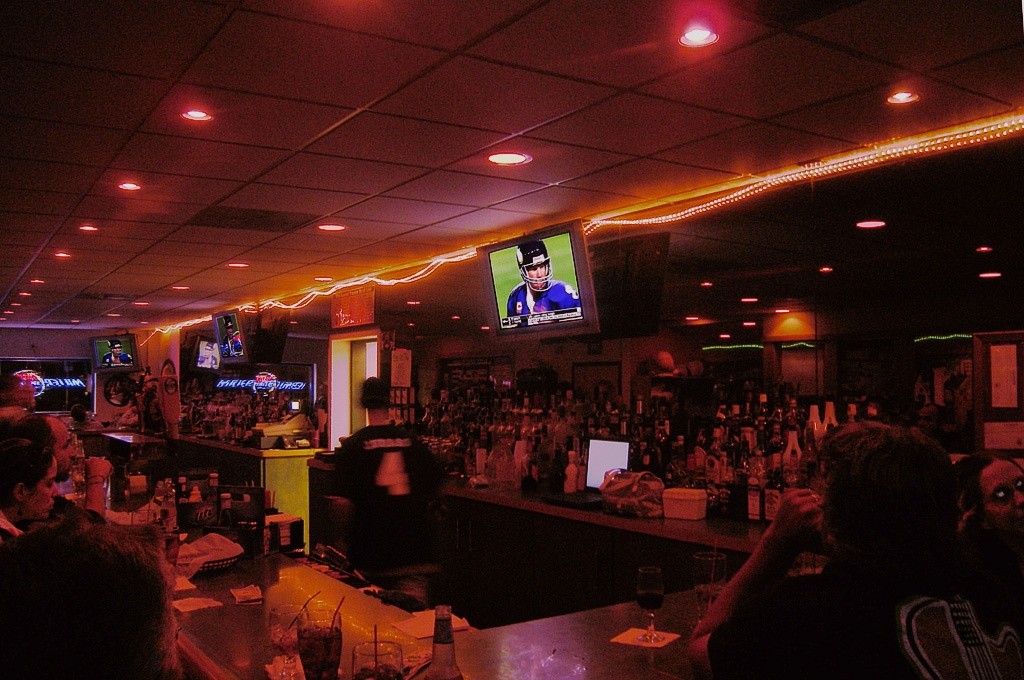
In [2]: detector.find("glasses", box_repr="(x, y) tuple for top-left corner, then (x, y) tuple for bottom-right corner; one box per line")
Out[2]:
(980, 476), (1024, 509)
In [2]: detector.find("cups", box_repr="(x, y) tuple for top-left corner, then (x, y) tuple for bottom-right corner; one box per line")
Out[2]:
(296, 610), (342, 680)
(72, 465), (85, 495)
(351, 641), (403, 680)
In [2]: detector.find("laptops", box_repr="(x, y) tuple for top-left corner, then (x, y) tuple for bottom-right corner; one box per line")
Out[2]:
(541, 438), (632, 508)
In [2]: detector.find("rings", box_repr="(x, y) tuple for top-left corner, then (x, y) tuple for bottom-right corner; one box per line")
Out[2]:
(810, 495), (814, 502)
(807, 488), (812, 494)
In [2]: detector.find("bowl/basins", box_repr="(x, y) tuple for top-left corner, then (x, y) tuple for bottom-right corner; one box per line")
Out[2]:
(663, 488), (706, 520)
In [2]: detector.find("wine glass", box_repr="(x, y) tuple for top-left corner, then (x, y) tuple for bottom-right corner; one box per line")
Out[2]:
(270, 605), (309, 680)
(689, 552), (725, 630)
(636, 567), (664, 643)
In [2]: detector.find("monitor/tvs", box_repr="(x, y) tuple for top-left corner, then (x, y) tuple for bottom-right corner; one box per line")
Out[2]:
(190, 336), (226, 376)
(475, 218), (601, 345)
(539, 232), (671, 342)
(289, 399), (303, 415)
(88, 334), (145, 375)
(211, 309), (249, 364)
(249, 308), (294, 364)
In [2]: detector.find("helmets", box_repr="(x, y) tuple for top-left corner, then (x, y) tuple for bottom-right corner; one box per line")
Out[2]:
(107, 340), (121, 347)
(223, 316), (233, 329)
(516, 241), (550, 269)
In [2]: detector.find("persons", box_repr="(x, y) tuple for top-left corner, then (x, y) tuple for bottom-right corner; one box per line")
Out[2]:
(318, 376), (448, 602)
(686, 420), (1024, 680)
(505, 240), (581, 324)
(220, 316), (242, 357)
(0, 375), (181, 680)
(102, 340), (133, 365)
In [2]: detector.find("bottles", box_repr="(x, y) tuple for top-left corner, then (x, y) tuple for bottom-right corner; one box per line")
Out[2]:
(153, 478), (176, 520)
(822, 402), (838, 437)
(747, 470), (763, 523)
(423, 605), (464, 680)
(218, 492), (232, 526)
(763, 454), (788, 526)
(188, 486), (202, 503)
(415, 389), (586, 495)
(588, 380), (804, 480)
(175, 476), (189, 503)
(846, 403), (857, 423)
(205, 472), (219, 525)
(809, 405), (822, 448)
(867, 402), (876, 420)
(804, 421), (818, 486)
(72, 434), (77, 449)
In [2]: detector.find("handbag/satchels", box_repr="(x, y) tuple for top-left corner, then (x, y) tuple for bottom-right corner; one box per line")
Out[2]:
(599, 467), (663, 516)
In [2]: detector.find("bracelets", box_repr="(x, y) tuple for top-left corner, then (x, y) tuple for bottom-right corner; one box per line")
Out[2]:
(86, 474), (104, 486)
